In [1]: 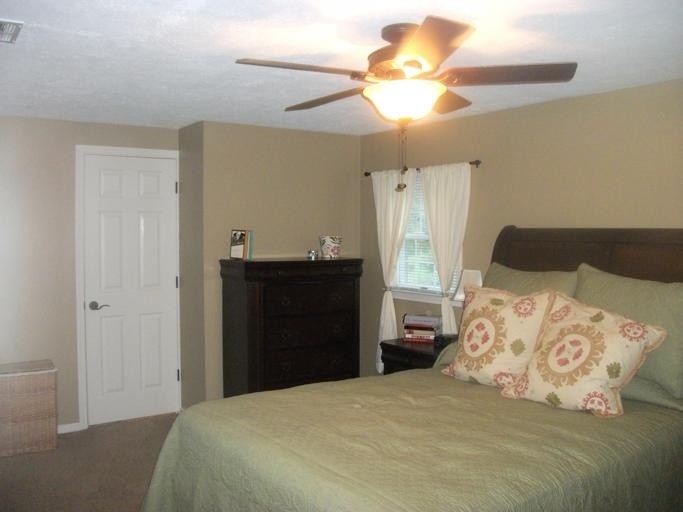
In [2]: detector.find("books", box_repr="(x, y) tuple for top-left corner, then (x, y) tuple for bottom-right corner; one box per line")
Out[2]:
(402, 314), (441, 343)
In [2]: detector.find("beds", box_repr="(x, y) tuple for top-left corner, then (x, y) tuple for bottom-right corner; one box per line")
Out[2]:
(145, 226), (681, 510)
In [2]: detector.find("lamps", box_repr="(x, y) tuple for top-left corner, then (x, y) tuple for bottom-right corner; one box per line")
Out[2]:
(361, 79), (447, 124)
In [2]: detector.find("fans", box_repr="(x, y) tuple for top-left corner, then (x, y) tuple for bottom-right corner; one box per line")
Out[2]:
(232, 14), (584, 116)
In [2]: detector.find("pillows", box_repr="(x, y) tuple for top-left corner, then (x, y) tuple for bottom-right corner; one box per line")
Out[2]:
(430, 260), (682, 419)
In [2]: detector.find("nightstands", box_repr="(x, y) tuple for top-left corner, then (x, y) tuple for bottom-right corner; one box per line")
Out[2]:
(379, 333), (458, 375)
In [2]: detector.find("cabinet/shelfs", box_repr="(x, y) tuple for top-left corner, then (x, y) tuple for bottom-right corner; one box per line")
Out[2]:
(218, 256), (363, 398)
